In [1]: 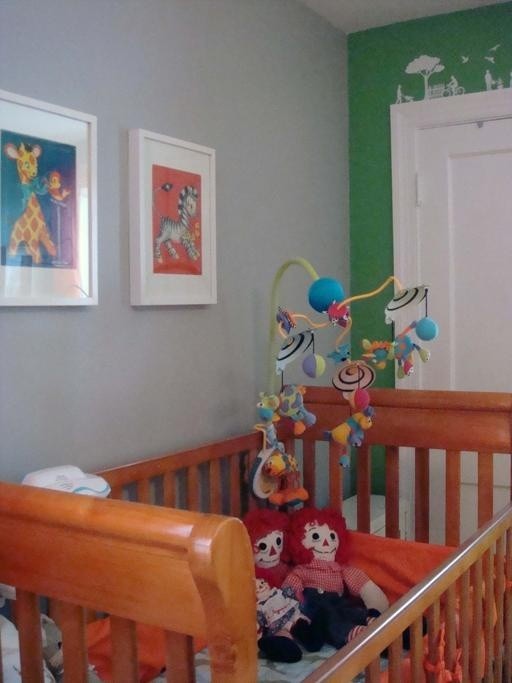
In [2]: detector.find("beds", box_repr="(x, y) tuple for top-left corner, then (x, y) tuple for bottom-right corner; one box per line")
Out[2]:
(0, 385), (512, 683)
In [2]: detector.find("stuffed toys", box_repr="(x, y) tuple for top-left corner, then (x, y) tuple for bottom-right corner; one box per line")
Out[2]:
(279, 505), (389, 647)
(239, 510), (313, 662)
(40, 612), (101, 683)
(248, 257), (438, 515)
